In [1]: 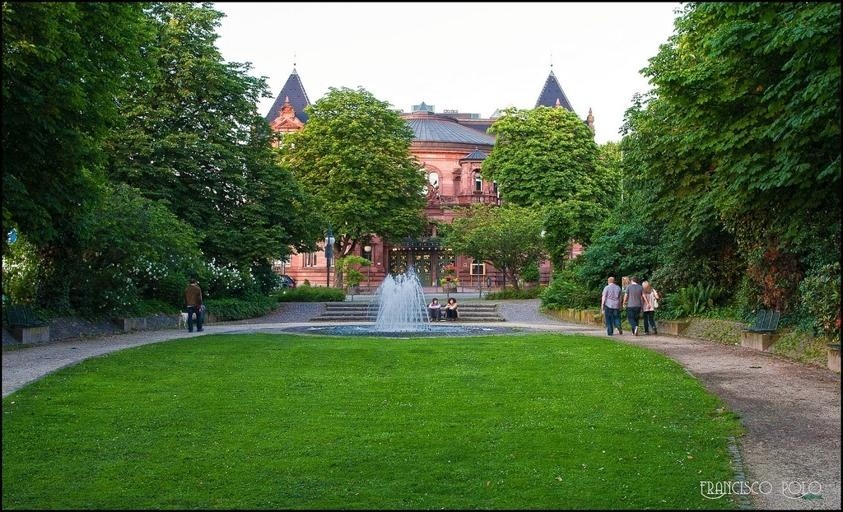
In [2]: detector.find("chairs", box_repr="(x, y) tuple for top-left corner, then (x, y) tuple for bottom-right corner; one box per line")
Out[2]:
(6, 305), (51, 346)
(742, 309), (780, 334)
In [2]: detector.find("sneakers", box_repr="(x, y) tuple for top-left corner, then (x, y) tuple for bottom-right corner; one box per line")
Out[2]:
(632, 326), (639, 336)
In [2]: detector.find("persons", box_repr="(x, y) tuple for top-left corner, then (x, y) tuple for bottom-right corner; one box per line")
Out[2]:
(639, 280), (659, 335)
(427, 297), (441, 322)
(183, 278), (204, 332)
(443, 297), (459, 322)
(622, 276), (643, 335)
(600, 276), (622, 336)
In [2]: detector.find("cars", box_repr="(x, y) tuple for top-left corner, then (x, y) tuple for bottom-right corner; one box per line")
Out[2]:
(226, 272), (296, 296)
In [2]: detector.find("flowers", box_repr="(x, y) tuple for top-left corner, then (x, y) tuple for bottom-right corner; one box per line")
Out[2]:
(439, 264), (459, 289)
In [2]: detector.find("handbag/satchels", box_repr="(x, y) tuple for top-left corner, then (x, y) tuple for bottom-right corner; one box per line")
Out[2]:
(653, 289), (658, 308)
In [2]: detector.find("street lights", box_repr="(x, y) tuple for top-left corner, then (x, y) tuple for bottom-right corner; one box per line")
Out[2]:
(323, 224), (335, 288)
(362, 233), (376, 284)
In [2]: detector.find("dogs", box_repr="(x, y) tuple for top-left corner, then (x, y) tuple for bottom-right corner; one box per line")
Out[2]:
(178, 305), (206, 330)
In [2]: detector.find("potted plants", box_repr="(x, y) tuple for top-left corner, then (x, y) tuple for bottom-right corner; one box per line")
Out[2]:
(342, 268), (363, 295)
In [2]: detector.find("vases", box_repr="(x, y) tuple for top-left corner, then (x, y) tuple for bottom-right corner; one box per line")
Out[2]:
(443, 287), (457, 293)
